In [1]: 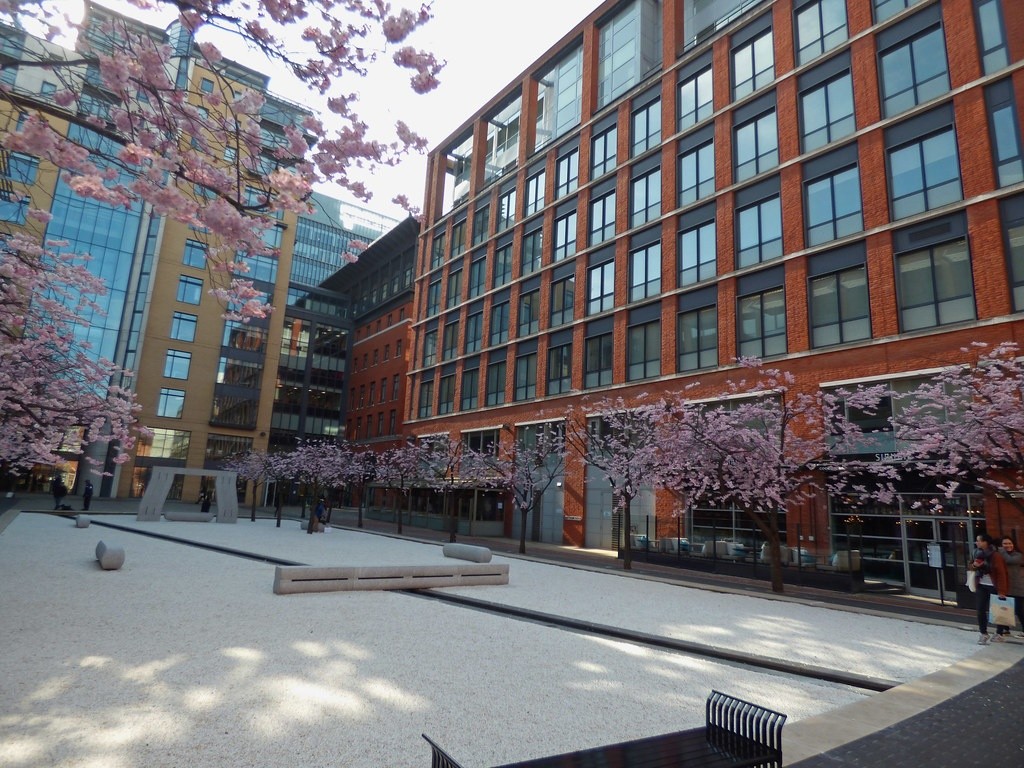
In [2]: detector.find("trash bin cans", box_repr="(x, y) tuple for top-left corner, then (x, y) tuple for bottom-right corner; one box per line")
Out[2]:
(324, 505), (331, 522)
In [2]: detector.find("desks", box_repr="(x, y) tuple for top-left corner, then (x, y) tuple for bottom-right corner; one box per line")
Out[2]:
(649, 540), (660, 548)
(802, 554), (832, 571)
(737, 547), (762, 558)
(680, 543), (704, 552)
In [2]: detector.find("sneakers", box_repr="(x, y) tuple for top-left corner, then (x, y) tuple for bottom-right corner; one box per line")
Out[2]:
(1015, 633), (1024, 638)
(990, 633), (1004, 642)
(976, 632), (990, 645)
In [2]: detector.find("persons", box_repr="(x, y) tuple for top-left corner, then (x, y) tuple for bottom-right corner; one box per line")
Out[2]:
(81, 480), (93, 511)
(312, 498), (327, 531)
(201, 496), (212, 513)
(274, 493), (279, 517)
(968, 532), (1024, 645)
(52, 475), (67, 512)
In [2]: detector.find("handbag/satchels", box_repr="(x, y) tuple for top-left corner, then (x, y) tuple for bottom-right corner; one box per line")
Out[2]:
(966, 571), (976, 593)
(988, 594), (1015, 627)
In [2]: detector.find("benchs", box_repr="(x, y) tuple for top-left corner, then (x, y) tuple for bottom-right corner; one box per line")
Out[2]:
(301, 520), (325, 531)
(96, 539), (125, 569)
(164, 512), (213, 522)
(76, 514), (90, 528)
(630, 534), (860, 572)
(421, 689), (787, 768)
(444, 542), (492, 563)
